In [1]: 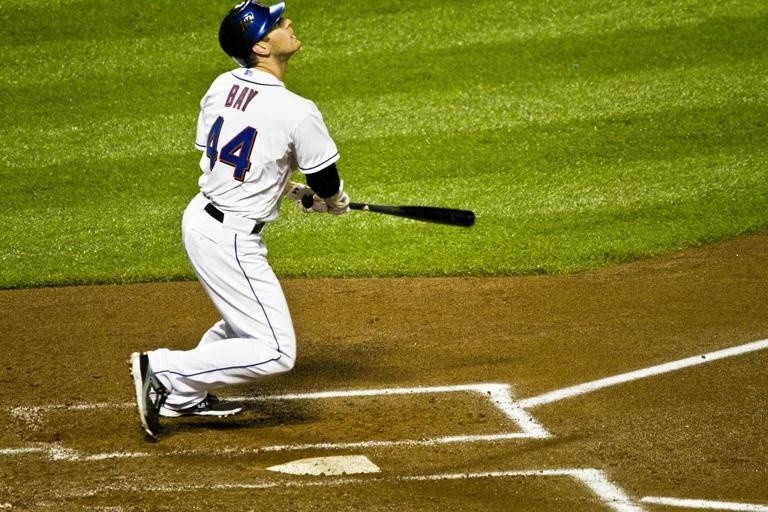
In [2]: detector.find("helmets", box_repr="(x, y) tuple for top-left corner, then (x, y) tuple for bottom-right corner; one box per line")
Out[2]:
(219, 1), (285, 67)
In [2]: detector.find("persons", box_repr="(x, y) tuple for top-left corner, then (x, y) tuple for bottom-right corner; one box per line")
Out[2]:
(127, 1), (350, 442)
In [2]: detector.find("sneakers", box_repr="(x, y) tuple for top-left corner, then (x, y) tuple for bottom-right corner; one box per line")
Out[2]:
(157, 392), (242, 418)
(129, 351), (169, 441)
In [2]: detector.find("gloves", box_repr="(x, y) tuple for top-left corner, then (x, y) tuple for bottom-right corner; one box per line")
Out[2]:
(287, 180), (351, 215)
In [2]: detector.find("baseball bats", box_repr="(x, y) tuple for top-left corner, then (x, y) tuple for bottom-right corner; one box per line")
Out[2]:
(302, 194), (474, 227)
